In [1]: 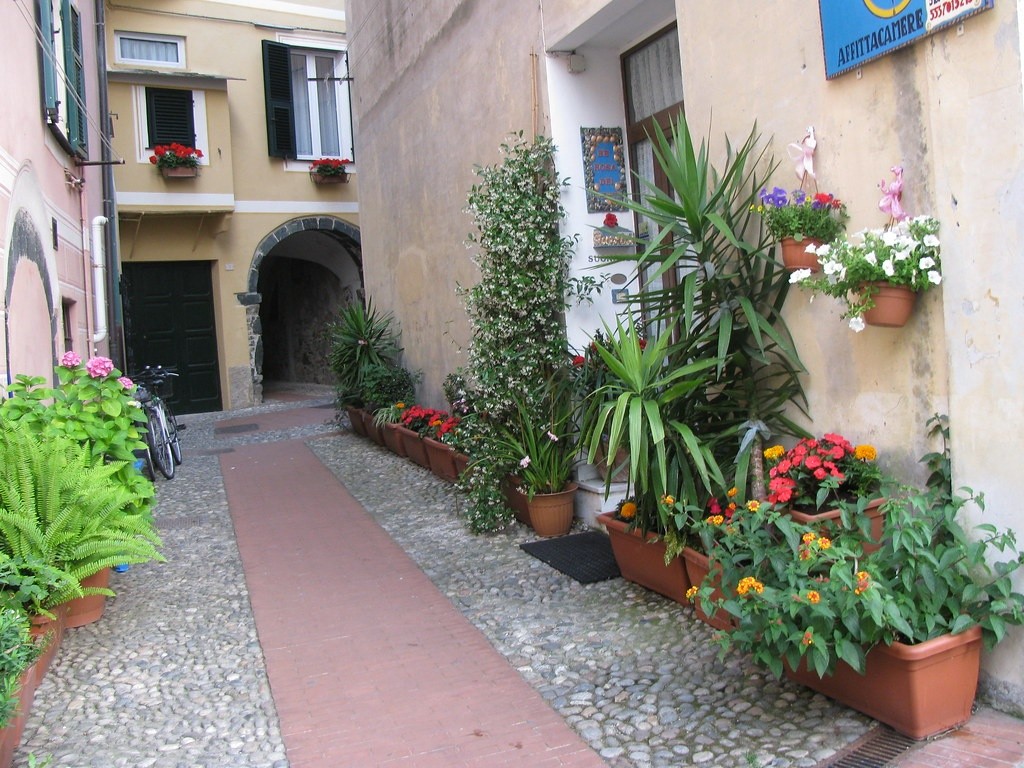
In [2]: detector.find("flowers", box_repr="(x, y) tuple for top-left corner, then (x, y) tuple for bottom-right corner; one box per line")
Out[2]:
(788, 214), (947, 333)
(614, 433), (1024, 681)
(148, 142), (203, 167)
(750, 185), (849, 239)
(381, 127), (569, 533)
(308, 158), (351, 175)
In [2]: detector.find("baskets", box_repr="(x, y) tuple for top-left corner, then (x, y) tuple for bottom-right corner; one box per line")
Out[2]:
(138, 375), (173, 399)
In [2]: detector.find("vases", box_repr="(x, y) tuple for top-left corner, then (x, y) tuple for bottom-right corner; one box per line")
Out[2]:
(781, 236), (821, 274)
(384, 421), (578, 537)
(859, 281), (917, 328)
(310, 172), (351, 184)
(162, 166), (197, 178)
(597, 495), (983, 741)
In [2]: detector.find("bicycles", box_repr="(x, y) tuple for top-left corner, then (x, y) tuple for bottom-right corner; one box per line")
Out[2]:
(130, 364), (186, 483)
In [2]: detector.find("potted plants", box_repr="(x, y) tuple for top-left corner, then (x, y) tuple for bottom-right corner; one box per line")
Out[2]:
(330, 294), (414, 448)
(0, 383), (164, 768)
